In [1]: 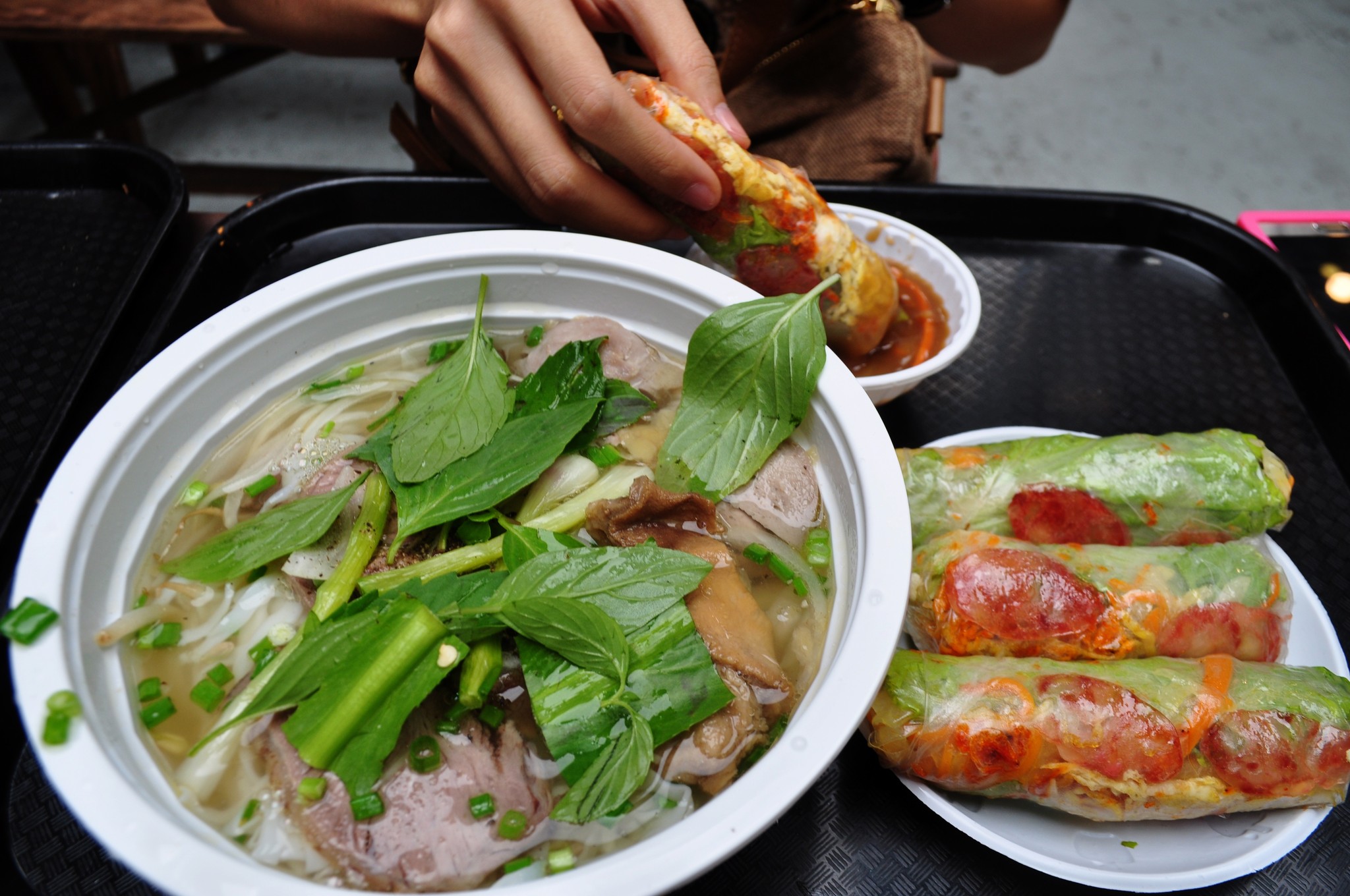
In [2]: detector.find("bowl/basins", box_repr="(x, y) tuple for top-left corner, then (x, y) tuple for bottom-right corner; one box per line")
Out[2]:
(687, 202), (982, 405)
(9, 229), (912, 896)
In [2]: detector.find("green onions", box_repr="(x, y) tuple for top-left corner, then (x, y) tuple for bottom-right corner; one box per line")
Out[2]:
(0, 325), (830, 878)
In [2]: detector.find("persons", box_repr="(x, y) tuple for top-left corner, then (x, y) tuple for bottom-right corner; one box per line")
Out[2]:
(205, 1), (1072, 240)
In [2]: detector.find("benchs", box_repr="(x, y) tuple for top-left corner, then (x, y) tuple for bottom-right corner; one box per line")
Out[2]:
(0, 0), (285, 146)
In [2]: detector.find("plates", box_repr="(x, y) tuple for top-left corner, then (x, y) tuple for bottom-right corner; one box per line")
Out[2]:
(887, 422), (1349, 893)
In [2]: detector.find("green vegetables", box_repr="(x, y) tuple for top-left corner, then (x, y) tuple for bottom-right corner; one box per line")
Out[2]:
(154, 272), (843, 824)
(891, 429), (1350, 757)
